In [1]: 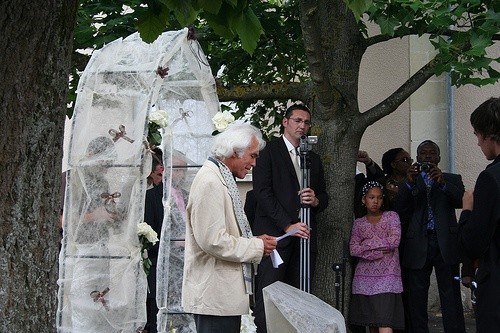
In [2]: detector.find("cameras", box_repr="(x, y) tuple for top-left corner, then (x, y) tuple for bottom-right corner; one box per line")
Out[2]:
(300, 135), (318, 153)
(418, 162), (435, 172)
(110, 206), (127, 235)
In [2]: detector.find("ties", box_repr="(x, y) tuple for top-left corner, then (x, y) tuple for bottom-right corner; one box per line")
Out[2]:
(293, 149), (301, 189)
(424, 174), (435, 229)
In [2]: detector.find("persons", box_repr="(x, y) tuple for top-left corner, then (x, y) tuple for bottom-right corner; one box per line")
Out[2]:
(182, 120), (279, 333)
(349, 181), (406, 333)
(457, 96), (500, 333)
(252, 104), (329, 333)
(353, 151), (383, 220)
(59, 137), (189, 333)
(403, 140), (467, 333)
(381, 148), (429, 333)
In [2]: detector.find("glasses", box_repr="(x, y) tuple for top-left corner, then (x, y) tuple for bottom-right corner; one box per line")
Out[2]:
(390, 157), (413, 164)
(288, 117), (311, 127)
